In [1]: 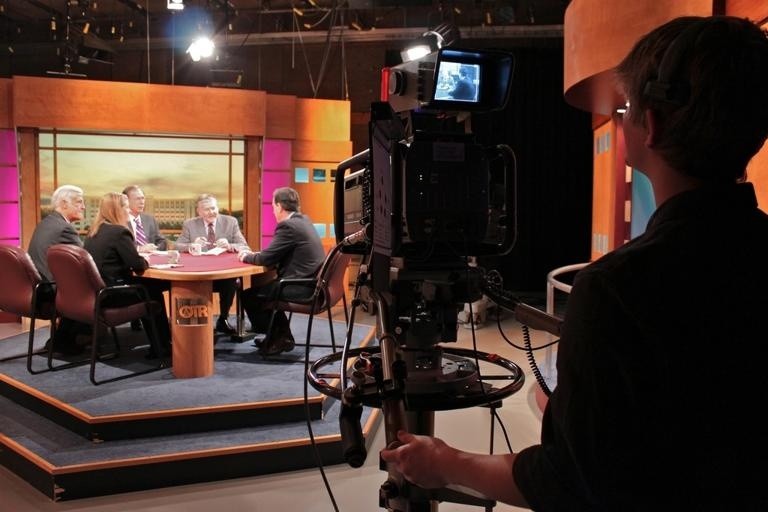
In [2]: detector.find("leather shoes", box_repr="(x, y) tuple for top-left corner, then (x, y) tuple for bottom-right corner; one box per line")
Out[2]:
(216, 318), (236, 333)
(259, 336), (295, 356)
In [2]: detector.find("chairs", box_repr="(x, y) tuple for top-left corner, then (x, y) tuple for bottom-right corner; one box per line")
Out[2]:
(0, 243), (102, 375)
(45, 241), (169, 386)
(252, 237), (355, 379)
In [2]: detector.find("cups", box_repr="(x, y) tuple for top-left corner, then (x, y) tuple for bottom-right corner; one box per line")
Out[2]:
(189, 244), (202, 255)
(167, 251), (179, 264)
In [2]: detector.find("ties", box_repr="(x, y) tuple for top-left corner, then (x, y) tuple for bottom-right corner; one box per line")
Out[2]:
(207, 223), (216, 247)
(134, 218), (148, 246)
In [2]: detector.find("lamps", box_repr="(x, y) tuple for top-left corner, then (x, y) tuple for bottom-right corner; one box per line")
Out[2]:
(397, 17), (465, 68)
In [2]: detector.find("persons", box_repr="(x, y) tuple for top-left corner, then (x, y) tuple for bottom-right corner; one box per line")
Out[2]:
(121, 185), (167, 253)
(174, 193), (251, 335)
(26, 184), (108, 358)
(379, 13), (768, 512)
(237, 186), (326, 355)
(448, 67), (476, 101)
(83, 192), (172, 363)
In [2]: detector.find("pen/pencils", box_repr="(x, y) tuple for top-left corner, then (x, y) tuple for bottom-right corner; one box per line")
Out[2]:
(206, 241), (216, 245)
(171, 265), (184, 268)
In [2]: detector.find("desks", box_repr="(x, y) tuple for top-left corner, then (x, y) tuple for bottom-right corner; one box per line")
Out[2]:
(130, 249), (281, 382)
